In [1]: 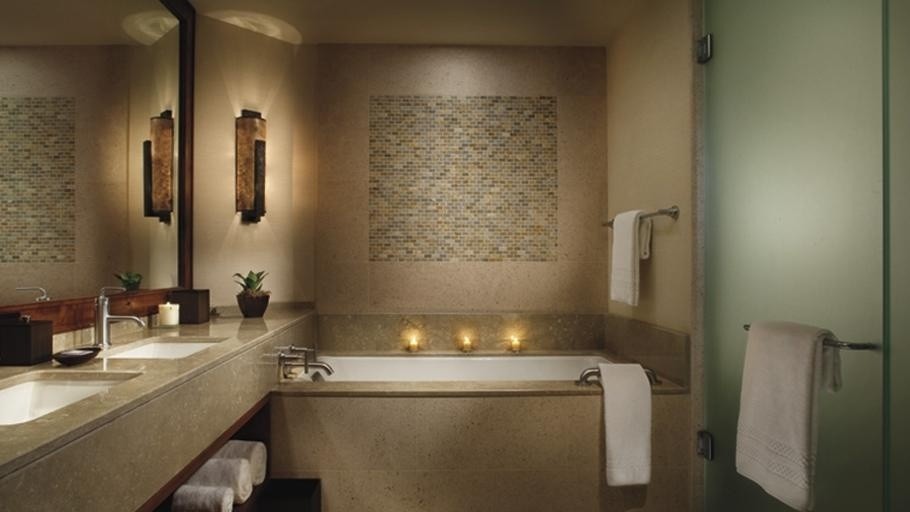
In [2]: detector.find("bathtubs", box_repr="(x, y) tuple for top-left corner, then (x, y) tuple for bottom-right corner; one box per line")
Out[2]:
(292, 355), (611, 383)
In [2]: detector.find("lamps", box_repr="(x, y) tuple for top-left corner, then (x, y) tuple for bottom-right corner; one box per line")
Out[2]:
(234, 109), (273, 225)
(144, 109), (175, 225)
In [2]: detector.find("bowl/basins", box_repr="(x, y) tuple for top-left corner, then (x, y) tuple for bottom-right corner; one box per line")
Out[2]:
(52, 347), (102, 366)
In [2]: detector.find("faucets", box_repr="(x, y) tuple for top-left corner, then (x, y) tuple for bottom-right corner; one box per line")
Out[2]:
(95, 286), (146, 348)
(16, 285), (50, 301)
(285, 360), (334, 376)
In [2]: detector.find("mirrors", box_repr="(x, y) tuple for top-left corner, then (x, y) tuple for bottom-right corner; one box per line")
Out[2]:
(0, 1), (196, 334)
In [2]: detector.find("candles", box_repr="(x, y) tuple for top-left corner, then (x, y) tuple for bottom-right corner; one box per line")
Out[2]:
(463, 342), (472, 351)
(158, 302), (180, 326)
(512, 341), (520, 352)
(408, 341), (418, 353)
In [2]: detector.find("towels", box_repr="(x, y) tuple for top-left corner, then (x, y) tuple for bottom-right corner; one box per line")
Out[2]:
(212, 438), (268, 490)
(610, 209), (652, 306)
(172, 484), (238, 512)
(598, 364), (652, 485)
(188, 457), (255, 501)
(735, 319), (841, 512)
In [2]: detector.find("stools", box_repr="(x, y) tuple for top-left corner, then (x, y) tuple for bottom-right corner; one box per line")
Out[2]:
(156, 478), (321, 511)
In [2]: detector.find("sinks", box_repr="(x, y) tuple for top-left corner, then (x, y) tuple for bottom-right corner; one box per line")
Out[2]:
(99, 336), (230, 360)
(0, 370), (141, 426)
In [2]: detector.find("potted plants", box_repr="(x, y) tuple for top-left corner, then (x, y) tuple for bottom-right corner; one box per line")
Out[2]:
(232, 270), (273, 318)
